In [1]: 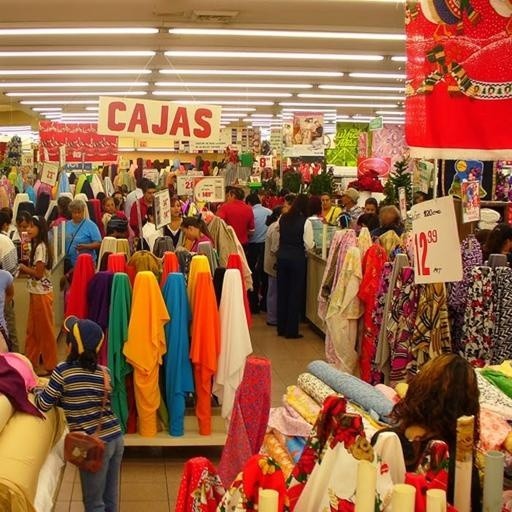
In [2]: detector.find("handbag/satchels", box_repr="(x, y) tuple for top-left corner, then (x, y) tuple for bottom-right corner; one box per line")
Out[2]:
(64, 256), (71, 272)
(64, 433), (105, 472)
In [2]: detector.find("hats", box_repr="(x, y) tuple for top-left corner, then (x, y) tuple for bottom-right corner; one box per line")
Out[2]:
(342, 189), (360, 204)
(64, 314), (105, 355)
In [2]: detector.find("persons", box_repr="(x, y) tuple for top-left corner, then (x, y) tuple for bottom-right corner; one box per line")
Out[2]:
(0, 166), (429, 377)
(372, 353), (482, 512)
(484, 225), (511, 266)
(33, 315), (125, 511)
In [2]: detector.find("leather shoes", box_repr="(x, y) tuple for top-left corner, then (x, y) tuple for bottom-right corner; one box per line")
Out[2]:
(286, 335), (303, 338)
